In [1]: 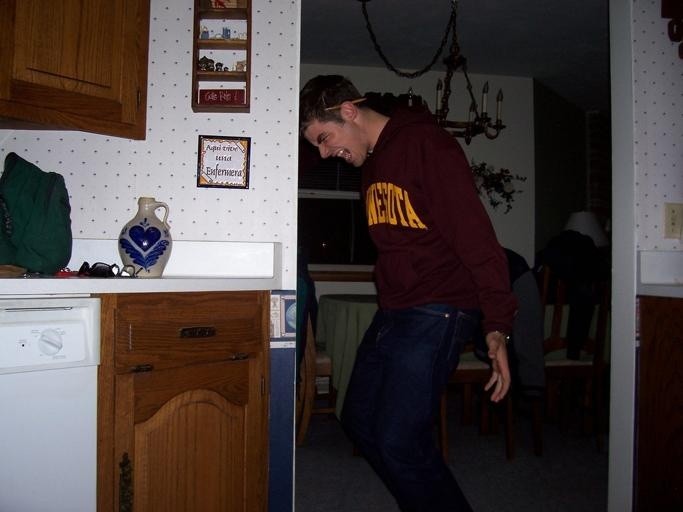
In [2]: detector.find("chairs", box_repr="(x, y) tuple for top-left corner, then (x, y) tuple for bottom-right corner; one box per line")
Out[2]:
(300, 268), (336, 436)
(442, 261), (606, 450)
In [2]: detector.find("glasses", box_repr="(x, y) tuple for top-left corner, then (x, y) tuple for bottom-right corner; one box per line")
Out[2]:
(109, 264), (144, 278)
(78, 261), (114, 278)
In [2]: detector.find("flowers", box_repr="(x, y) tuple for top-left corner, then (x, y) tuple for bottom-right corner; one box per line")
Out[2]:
(472, 159), (527, 213)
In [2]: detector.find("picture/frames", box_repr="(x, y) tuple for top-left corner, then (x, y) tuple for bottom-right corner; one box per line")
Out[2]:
(197, 135), (252, 189)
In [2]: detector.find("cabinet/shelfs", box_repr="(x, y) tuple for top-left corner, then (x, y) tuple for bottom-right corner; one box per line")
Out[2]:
(191, 0), (254, 113)
(100, 292), (271, 512)
(0, 0), (153, 140)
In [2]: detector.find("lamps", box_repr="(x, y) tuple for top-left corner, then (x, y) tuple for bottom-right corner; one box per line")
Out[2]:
(407, 0), (507, 146)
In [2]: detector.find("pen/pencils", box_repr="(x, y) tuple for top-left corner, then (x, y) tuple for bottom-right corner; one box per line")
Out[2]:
(325, 97), (367, 111)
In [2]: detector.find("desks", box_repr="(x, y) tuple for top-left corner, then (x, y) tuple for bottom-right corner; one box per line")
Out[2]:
(320, 294), (383, 322)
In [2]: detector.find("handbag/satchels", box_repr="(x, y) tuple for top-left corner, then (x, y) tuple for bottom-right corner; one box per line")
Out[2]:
(0, 152), (74, 274)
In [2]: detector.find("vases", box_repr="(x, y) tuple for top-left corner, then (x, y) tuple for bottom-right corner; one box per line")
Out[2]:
(116, 198), (174, 278)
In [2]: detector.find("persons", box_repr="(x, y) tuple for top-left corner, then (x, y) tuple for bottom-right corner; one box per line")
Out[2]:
(299, 74), (520, 512)
